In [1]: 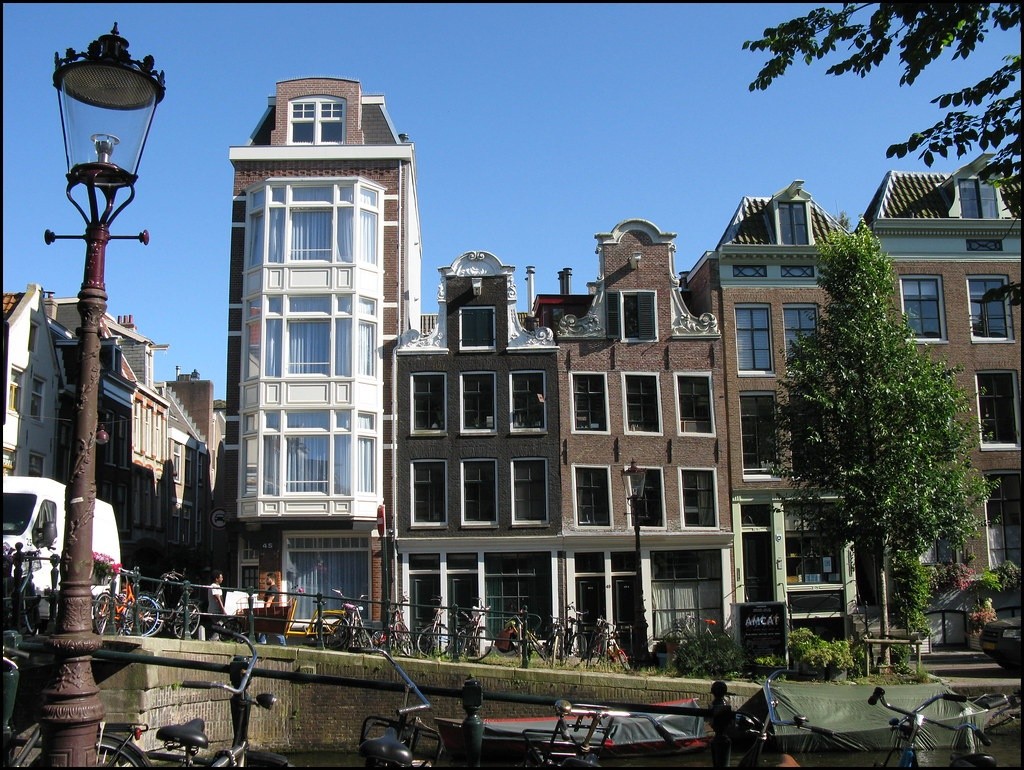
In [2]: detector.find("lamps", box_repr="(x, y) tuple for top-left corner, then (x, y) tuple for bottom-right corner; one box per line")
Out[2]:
(94, 423), (110, 445)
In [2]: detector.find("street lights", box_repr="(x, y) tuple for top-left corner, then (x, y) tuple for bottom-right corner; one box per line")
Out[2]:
(41, 18), (171, 770)
(621, 458), (650, 653)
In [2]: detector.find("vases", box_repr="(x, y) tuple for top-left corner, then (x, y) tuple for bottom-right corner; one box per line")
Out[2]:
(969, 627), (989, 650)
(91, 566), (109, 585)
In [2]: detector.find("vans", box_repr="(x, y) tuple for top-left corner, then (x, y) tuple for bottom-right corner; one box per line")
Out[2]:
(2, 475), (122, 621)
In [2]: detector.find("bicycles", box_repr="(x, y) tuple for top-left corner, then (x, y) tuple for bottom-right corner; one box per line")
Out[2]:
(332, 588), (414, 656)
(157, 623), (297, 770)
(871, 693), (969, 770)
(524, 700), (679, 769)
(414, 595), (455, 659)
(868, 686), (1002, 770)
(661, 615), (732, 644)
(505, 604), (630, 668)
(88, 557), (202, 636)
(0, 550), (43, 636)
(0, 646), (157, 770)
(355, 647), (444, 770)
(734, 669), (833, 770)
(458, 597), (496, 662)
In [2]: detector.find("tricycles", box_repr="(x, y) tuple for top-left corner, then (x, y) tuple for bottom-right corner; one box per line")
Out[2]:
(233, 585), (350, 648)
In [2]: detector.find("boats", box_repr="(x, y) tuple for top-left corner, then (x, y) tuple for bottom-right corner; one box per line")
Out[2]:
(432, 696), (717, 761)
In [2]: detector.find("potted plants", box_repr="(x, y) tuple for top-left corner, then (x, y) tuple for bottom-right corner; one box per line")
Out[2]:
(754, 626), (855, 681)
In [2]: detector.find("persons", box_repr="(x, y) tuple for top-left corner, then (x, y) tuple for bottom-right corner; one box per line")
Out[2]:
(258, 572), (287, 646)
(208, 569), (229, 624)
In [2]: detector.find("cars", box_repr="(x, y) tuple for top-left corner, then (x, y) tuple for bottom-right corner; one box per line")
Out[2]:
(978, 615), (1024, 671)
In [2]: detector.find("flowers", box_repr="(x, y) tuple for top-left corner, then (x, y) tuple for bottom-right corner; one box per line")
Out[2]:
(92, 551), (123, 584)
(969, 597), (997, 632)
(937, 564), (975, 592)
